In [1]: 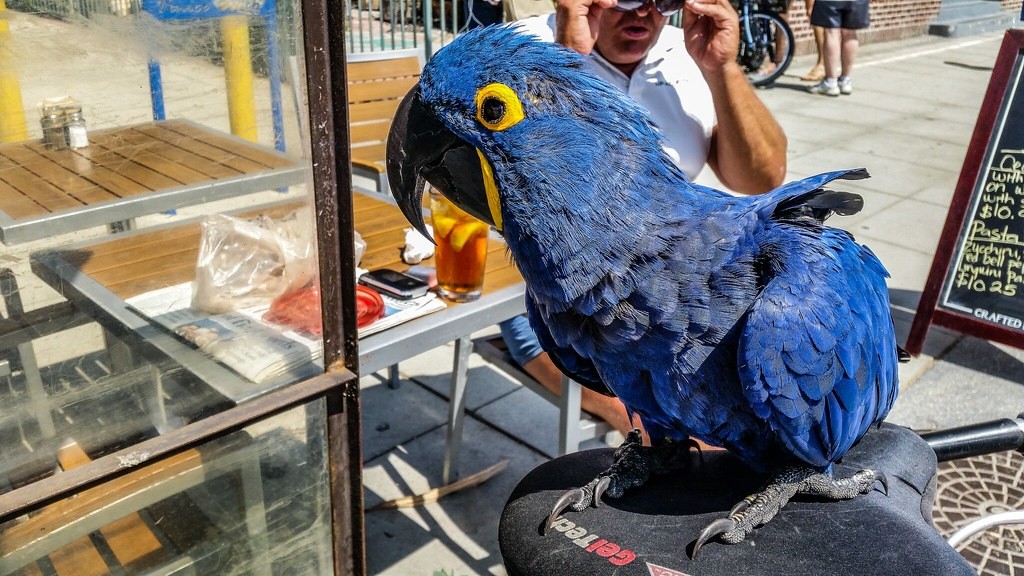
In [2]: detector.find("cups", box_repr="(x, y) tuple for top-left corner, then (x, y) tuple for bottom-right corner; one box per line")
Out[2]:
(429, 186), (491, 303)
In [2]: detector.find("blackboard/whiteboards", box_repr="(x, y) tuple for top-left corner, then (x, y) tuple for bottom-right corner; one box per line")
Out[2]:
(927, 30), (1024, 352)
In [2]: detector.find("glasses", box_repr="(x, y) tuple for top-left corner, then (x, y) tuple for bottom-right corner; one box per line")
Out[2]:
(613, 0), (682, 17)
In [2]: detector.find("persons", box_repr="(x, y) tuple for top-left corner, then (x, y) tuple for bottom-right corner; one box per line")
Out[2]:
(800, 0), (870, 96)
(499, 0), (788, 452)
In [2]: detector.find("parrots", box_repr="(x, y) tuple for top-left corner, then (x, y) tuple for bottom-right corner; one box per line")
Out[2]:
(382, 18), (901, 565)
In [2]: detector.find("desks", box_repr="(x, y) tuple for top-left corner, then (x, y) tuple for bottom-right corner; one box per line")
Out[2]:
(31, 185), (527, 576)
(0, 118), (311, 377)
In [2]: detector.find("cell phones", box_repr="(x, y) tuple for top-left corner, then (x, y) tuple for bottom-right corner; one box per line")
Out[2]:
(359, 269), (430, 299)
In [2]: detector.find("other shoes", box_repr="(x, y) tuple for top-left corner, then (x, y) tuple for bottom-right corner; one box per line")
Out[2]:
(801, 68), (825, 81)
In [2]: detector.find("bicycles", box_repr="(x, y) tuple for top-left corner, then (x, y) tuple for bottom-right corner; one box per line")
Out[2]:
(731, 0), (797, 88)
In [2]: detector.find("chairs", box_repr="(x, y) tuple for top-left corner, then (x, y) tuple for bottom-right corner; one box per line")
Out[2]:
(289, 48), (426, 194)
(442, 334), (614, 486)
(0, 267), (271, 576)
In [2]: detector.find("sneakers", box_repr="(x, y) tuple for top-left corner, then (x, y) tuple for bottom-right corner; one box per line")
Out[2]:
(805, 77), (852, 96)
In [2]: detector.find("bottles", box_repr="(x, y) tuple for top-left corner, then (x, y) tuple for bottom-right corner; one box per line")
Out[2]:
(40, 103), (90, 150)
